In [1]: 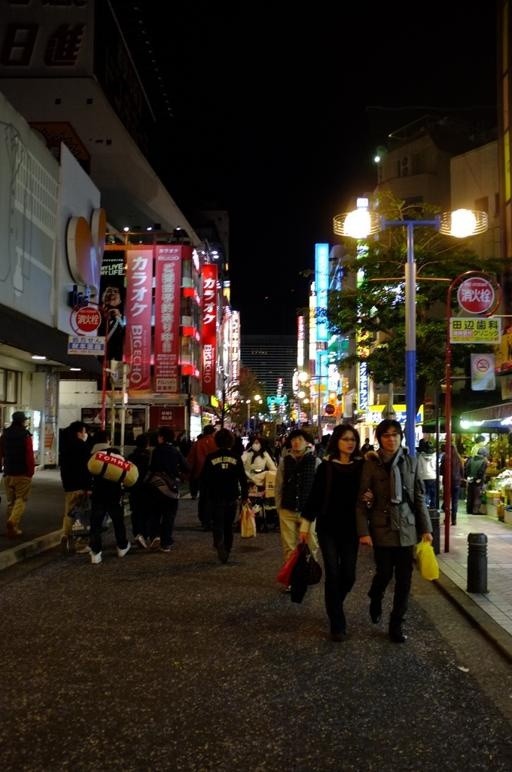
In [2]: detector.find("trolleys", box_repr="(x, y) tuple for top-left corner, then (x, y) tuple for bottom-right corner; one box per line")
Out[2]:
(248, 470), (280, 533)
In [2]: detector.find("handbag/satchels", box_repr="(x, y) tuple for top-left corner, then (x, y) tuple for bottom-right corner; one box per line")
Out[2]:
(416, 538), (439, 581)
(241, 504), (321, 604)
(87, 451), (139, 487)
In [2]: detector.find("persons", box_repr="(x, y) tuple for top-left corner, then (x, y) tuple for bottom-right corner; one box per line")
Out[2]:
(361, 438), (374, 457)
(416, 436), (486, 526)
(59, 420), (190, 565)
(193, 428), (249, 564)
(361, 419), (434, 643)
(274, 429), (322, 594)
(191, 418), (331, 522)
(1, 412), (35, 537)
(299, 422), (373, 643)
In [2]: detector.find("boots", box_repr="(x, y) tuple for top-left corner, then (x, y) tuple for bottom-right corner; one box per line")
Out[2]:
(332, 599), (408, 642)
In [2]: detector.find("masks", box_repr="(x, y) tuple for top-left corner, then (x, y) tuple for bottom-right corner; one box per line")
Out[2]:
(82, 433), (87, 442)
(250, 444), (261, 452)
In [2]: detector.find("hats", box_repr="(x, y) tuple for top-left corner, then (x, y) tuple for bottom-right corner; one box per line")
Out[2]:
(203, 424), (216, 437)
(12, 412), (27, 421)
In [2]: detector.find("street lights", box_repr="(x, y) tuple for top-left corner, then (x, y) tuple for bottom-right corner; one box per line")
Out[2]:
(333, 198), (488, 479)
(299, 371), (333, 442)
(221, 385), (263, 430)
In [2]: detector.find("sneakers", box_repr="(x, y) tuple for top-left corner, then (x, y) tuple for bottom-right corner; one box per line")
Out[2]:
(6, 520), (171, 564)
